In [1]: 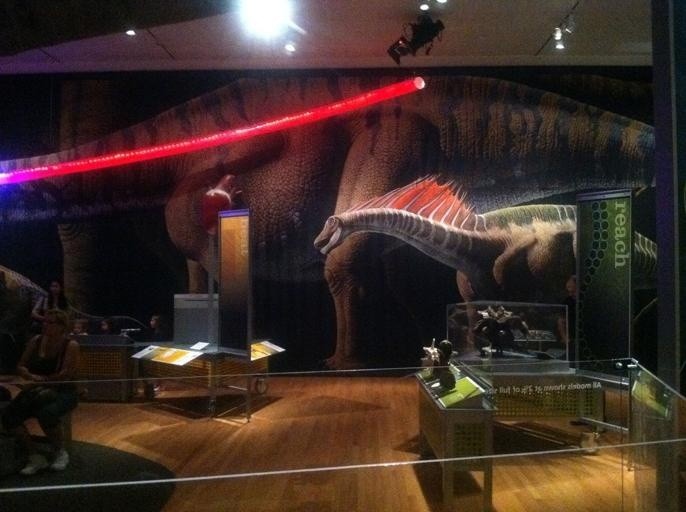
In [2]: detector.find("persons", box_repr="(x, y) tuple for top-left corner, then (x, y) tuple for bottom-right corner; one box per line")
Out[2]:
(150, 314), (164, 339)
(30, 282), (73, 331)
(70, 321), (90, 335)
(101, 320), (112, 335)
(562, 275), (577, 329)
(0, 308), (83, 477)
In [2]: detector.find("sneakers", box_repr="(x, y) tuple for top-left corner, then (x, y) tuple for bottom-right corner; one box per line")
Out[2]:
(20, 454), (48, 475)
(50, 448), (70, 471)
